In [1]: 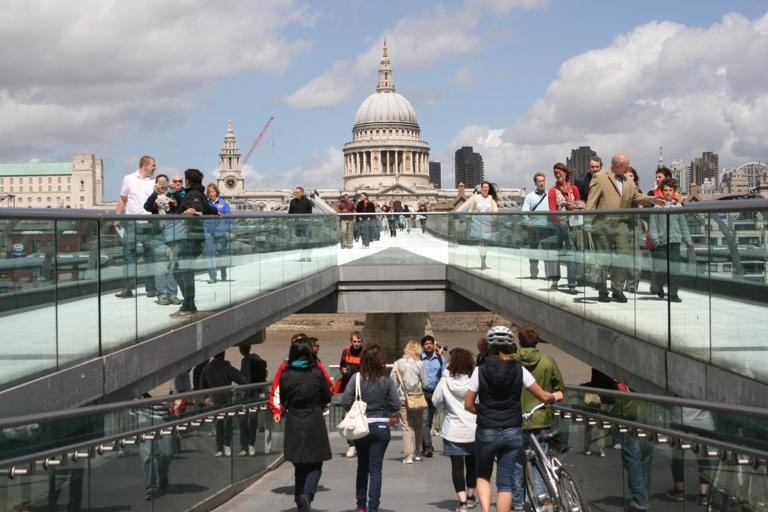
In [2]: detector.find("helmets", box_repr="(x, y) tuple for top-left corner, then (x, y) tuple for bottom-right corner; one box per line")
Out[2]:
(484, 325), (515, 345)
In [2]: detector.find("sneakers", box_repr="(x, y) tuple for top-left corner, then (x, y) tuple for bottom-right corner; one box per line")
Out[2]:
(457, 496), (477, 512)
(215, 444), (257, 458)
(207, 273), (226, 283)
(294, 498), (313, 511)
(168, 308), (195, 318)
(344, 445), (358, 460)
(145, 491), (160, 501)
(115, 288), (133, 299)
(157, 294), (181, 306)
(629, 486), (712, 511)
(401, 446), (435, 464)
(529, 272), (683, 307)
(145, 287), (157, 298)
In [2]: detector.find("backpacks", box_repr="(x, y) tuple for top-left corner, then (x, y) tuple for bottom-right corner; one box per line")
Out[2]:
(250, 361), (268, 385)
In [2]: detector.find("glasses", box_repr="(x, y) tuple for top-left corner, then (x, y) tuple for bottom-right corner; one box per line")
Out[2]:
(173, 180), (182, 183)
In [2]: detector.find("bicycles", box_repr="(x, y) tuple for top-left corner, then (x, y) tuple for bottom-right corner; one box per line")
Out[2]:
(522, 400), (591, 511)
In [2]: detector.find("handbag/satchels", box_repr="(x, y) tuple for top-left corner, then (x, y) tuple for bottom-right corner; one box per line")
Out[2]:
(573, 250), (603, 285)
(336, 400), (372, 440)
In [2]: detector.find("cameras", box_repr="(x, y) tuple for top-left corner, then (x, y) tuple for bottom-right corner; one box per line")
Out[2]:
(159, 181), (168, 188)
(437, 345), (447, 351)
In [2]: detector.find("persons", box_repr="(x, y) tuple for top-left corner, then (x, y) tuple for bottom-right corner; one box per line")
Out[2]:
(110, 155), (232, 319)
(129, 391), (188, 501)
(662, 437), (711, 506)
(620, 433), (655, 510)
(287, 186), (314, 263)
(520, 153), (699, 305)
(453, 182), (499, 270)
(335, 193), (428, 250)
